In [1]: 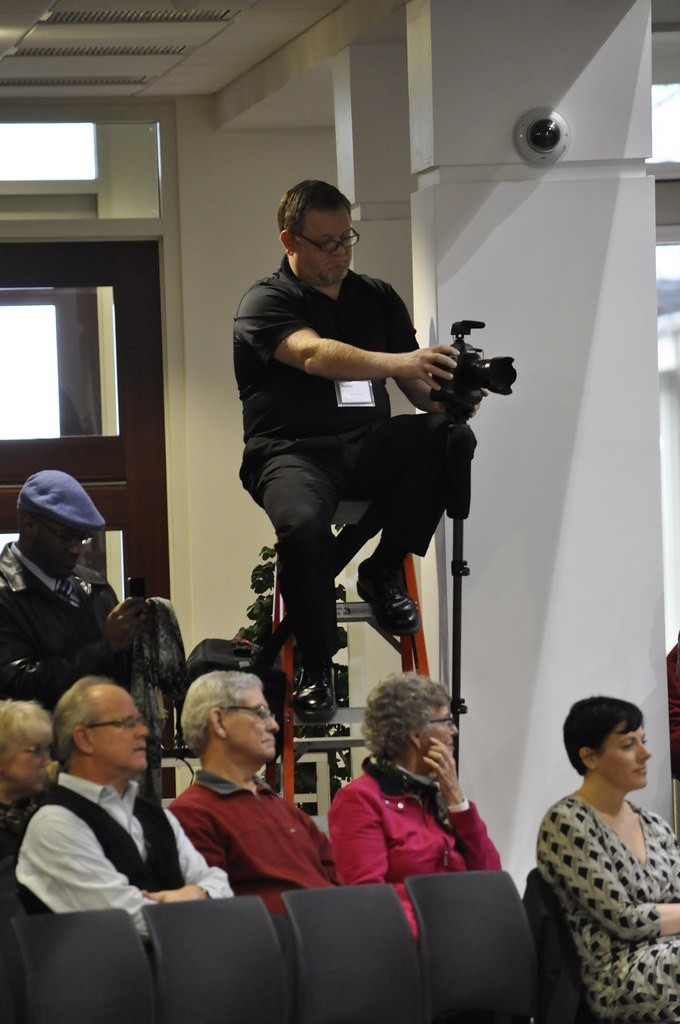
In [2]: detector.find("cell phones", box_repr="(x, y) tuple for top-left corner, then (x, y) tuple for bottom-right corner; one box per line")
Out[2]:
(129, 576), (146, 618)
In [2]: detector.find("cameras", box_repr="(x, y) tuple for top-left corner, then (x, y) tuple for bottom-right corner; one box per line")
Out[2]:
(429, 339), (517, 405)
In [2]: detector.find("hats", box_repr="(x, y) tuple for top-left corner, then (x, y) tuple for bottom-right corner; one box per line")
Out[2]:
(17, 469), (106, 533)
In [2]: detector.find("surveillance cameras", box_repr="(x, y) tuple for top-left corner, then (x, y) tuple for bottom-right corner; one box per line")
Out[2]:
(515, 107), (571, 164)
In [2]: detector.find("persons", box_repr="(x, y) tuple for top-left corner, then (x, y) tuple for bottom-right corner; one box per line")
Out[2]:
(535, 696), (680, 1024)
(0, 470), (502, 944)
(232, 179), (488, 721)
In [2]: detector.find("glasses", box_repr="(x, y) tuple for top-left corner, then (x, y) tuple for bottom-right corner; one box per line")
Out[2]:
(38, 518), (94, 546)
(220, 704), (277, 721)
(85, 712), (148, 730)
(429, 713), (455, 728)
(21, 742), (55, 757)
(295, 227), (361, 251)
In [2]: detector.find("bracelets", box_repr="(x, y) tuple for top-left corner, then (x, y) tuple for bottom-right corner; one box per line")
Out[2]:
(200, 886), (212, 900)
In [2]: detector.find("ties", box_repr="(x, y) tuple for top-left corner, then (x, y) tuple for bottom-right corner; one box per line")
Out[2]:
(53, 578), (82, 608)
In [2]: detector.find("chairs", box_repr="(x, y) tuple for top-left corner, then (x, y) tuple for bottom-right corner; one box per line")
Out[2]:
(0, 846), (680, 1024)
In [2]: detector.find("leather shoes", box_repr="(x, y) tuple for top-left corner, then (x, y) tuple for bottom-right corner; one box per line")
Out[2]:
(357, 557), (421, 634)
(292, 644), (337, 721)
